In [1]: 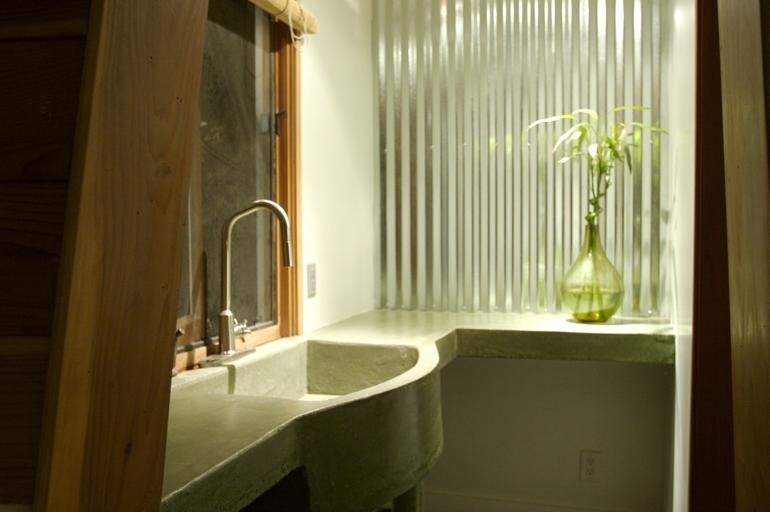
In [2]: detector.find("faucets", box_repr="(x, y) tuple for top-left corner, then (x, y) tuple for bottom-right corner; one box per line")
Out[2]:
(219, 199), (293, 354)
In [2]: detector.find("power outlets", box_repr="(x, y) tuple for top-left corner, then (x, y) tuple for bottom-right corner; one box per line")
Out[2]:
(580, 449), (600, 484)
(308, 263), (316, 298)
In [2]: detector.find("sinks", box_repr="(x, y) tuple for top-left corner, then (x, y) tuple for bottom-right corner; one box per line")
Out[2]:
(189, 339), (443, 510)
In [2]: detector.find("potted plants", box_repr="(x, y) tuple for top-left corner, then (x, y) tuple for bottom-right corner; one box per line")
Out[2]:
(527, 105), (671, 322)
